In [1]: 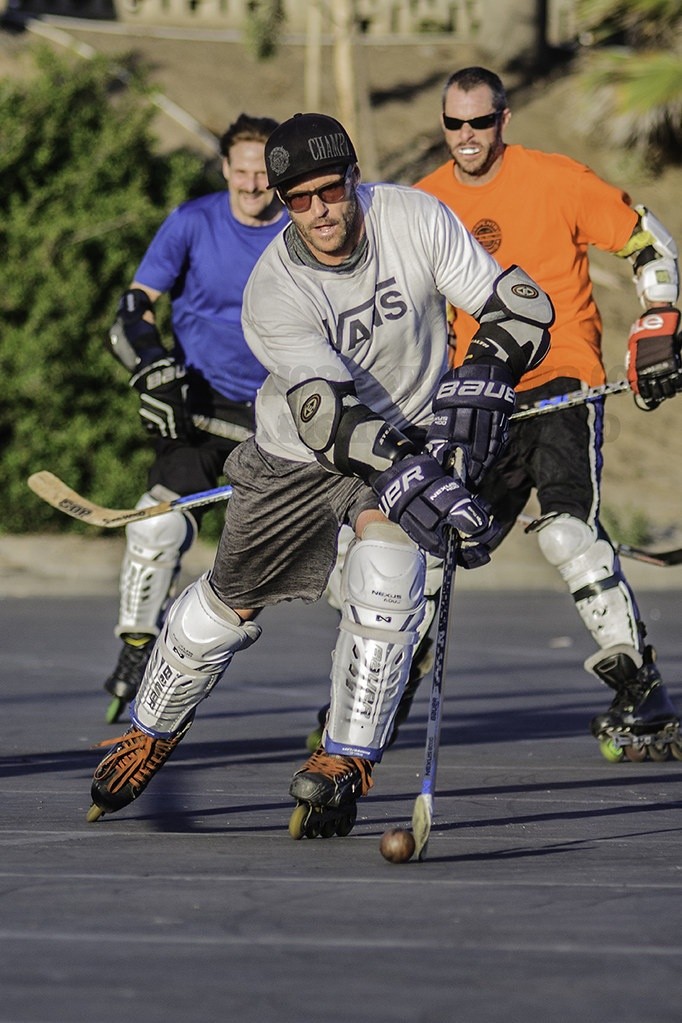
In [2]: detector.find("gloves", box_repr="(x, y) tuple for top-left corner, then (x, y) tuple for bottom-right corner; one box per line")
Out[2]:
(625, 307), (682, 412)
(422, 364), (516, 492)
(128, 356), (212, 441)
(373, 454), (491, 569)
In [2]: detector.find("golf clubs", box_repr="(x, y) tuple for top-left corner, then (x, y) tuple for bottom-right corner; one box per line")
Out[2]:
(184, 413), (680, 570)
(24, 355), (631, 529)
(411, 447), (469, 854)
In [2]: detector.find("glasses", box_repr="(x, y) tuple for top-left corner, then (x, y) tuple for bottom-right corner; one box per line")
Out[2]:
(278, 164), (353, 213)
(443, 110), (503, 130)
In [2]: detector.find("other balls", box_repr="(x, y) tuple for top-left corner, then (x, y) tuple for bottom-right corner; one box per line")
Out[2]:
(378, 822), (417, 866)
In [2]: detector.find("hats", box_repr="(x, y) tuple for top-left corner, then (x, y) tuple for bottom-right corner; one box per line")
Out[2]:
(264, 112), (357, 191)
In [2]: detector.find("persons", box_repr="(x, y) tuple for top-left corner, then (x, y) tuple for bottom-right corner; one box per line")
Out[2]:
(307, 68), (682, 758)
(98, 115), (297, 728)
(84, 112), (555, 842)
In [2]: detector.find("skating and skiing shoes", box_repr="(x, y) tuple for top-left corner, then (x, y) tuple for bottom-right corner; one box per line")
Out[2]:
(306, 703), (329, 752)
(105, 634), (155, 723)
(288, 736), (378, 840)
(86, 709), (197, 823)
(582, 643), (682, 763)
